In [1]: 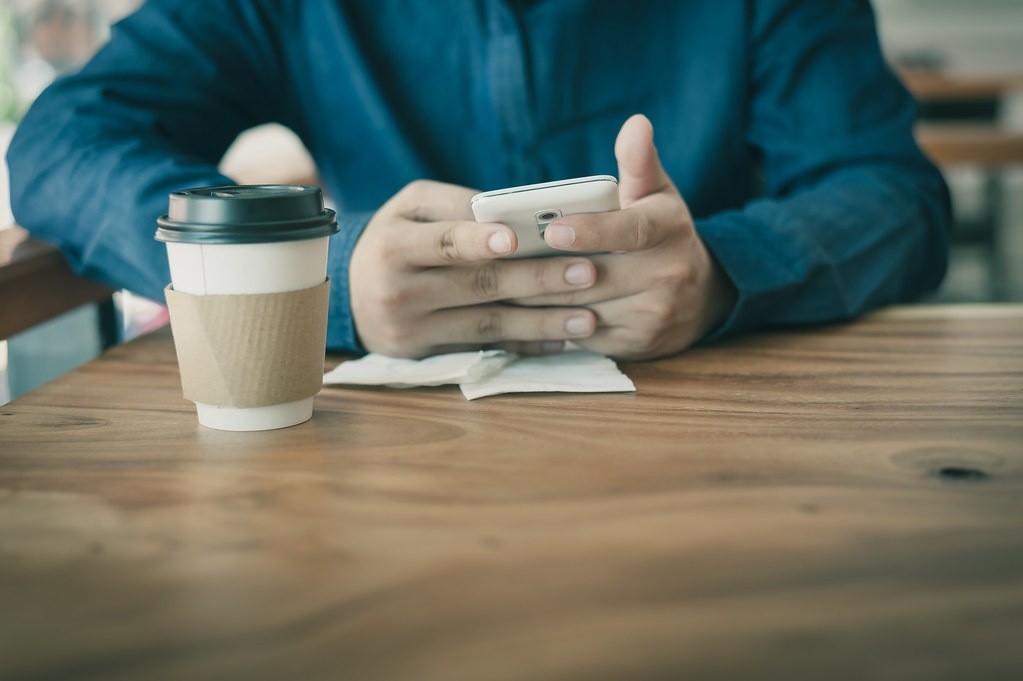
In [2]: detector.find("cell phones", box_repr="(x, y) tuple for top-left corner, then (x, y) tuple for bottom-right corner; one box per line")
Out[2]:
(472, 175), (620, 258)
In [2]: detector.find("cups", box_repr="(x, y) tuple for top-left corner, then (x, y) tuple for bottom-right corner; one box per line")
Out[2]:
(154, 185), (341, 432)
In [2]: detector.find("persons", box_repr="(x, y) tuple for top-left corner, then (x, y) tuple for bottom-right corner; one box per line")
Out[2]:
(5, 0), (955, 359)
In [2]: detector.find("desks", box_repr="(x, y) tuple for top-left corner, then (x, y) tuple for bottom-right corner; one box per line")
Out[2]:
(0, 303), (1023, 681)
